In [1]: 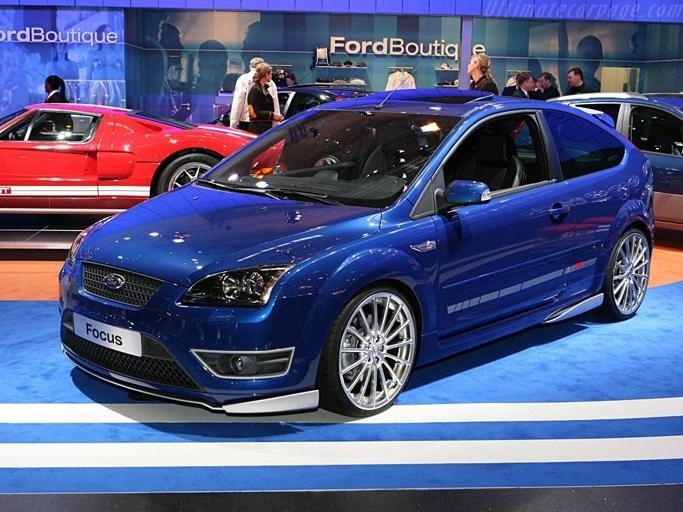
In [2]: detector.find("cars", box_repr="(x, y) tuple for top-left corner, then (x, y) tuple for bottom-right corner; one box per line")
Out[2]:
(219, 81), (373, 137)
(515, 82), (682, 235)
(55, 82), (659, 425)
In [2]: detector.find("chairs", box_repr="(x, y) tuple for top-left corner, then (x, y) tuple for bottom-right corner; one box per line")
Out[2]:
(450, 141), (526, 191)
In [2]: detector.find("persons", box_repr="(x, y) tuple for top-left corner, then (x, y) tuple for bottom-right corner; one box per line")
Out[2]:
(45, 75), (73, 131)
(286, 73), (296, 85)
(230, 58), (284, 134)
(467, 53), (499, 97)
(501, 68), (594, 101)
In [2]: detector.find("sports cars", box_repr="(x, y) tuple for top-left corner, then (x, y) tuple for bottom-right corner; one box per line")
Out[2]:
(1, 100), (285, 219)
